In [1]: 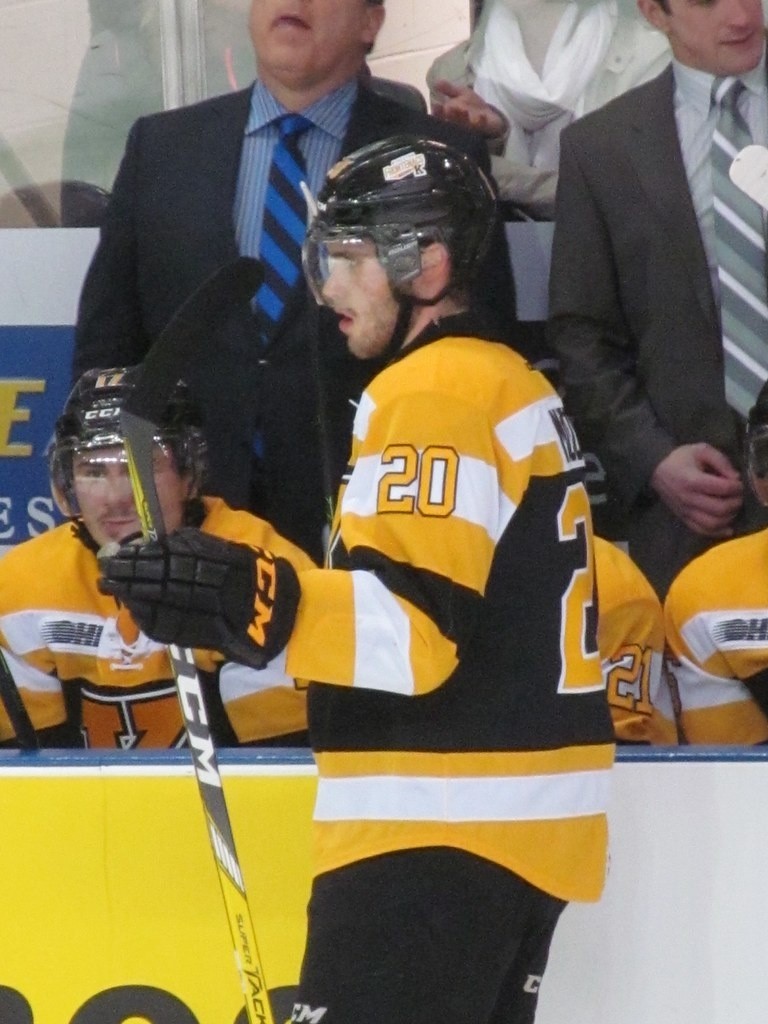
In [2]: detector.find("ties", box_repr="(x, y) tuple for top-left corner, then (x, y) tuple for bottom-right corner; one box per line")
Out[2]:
(709, 77), (767, 416)
(243, 113), (318, 460)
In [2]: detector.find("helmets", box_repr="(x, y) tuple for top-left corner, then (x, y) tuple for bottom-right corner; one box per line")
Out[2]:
(49, 367), (212, 519)
(301, 135), (498, 308)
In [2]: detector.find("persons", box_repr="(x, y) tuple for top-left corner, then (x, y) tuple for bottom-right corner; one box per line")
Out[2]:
(550, 0), (768, 607)
(425, 0), (676, 370)
(589, 532), (681, 749)
(89, 134), (615, 1024)
(661, 377), (768, 752)
(0, 363), (320, 750)
(69, 0), (522, 568)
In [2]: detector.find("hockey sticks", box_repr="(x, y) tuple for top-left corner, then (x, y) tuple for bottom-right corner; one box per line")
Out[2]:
(114, 415), (279, 1023)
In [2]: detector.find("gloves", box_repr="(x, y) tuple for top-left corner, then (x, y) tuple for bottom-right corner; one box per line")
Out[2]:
(95, 526), (303, 672)
